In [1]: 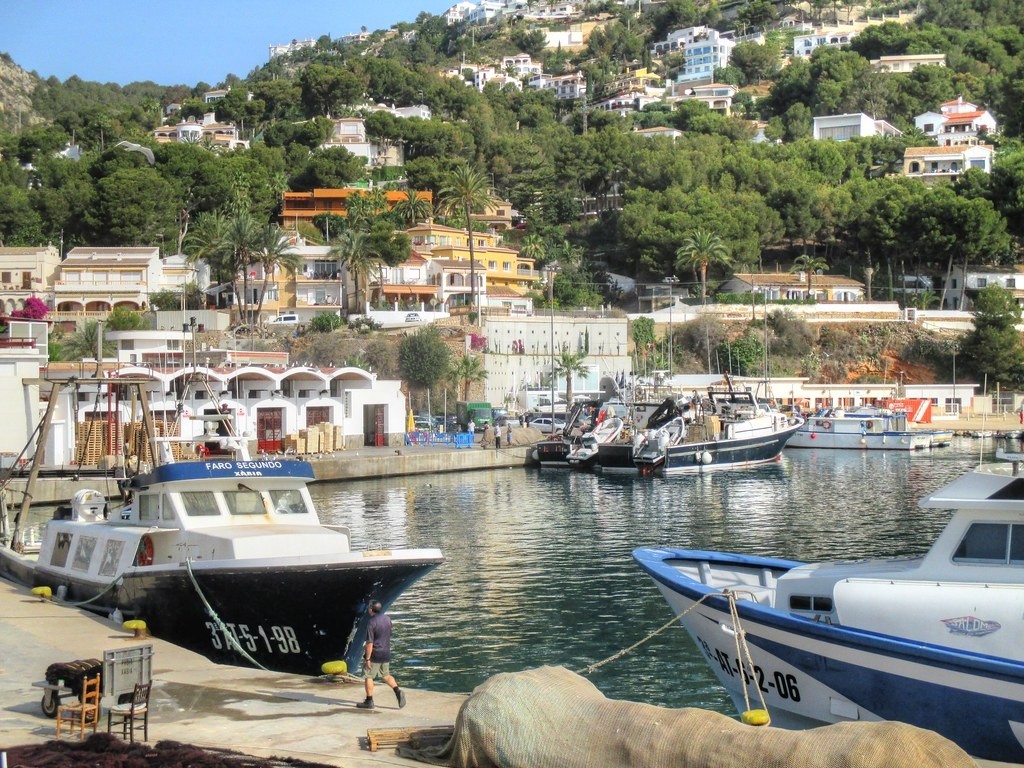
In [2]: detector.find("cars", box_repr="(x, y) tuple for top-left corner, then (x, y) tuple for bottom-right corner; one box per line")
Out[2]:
(408, 413), (458, 434)
(527, 417), (567, 434)
(405, 313), (422, 323)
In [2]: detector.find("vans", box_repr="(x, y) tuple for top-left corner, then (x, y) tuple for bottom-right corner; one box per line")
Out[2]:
(271, 314), (299, 324)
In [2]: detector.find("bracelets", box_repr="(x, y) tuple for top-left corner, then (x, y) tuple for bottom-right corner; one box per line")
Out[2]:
(366, 659), (370, 661)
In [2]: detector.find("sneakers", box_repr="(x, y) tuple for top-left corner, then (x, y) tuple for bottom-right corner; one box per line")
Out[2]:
(399, 691), (407, 708)
(357, 700), (374, 708)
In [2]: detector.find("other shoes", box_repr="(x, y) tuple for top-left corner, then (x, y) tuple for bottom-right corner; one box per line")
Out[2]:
(508, 445), (512, 448)
(506, 444), (509, 446)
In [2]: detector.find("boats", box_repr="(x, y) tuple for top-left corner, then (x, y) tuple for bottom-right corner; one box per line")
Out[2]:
(917, 458), (1024, 509)
(0, 315), (446, 677)
(630, 499), (1024, 764)
(521, 281), (1024, 484)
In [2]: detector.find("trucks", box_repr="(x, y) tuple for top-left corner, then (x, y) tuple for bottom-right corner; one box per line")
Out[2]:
(491, 406), (509, 427)
(456, 401), (494, 433)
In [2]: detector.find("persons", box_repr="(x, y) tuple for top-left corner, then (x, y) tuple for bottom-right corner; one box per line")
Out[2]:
(467, 420), (475, 435)
(484, 421), (490, 429)
(495, 423), (501, 448)
(357, 600), (406, 709)
(507, 423), (512, 446)
(124, 439), (130, 459)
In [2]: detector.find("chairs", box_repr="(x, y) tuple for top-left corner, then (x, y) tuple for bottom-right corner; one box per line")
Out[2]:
(106, 680), (153, 743)
(57, 673), (101, 740)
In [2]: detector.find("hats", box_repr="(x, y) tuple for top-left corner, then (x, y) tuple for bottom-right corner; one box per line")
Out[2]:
(484, 421), (488, 424)
